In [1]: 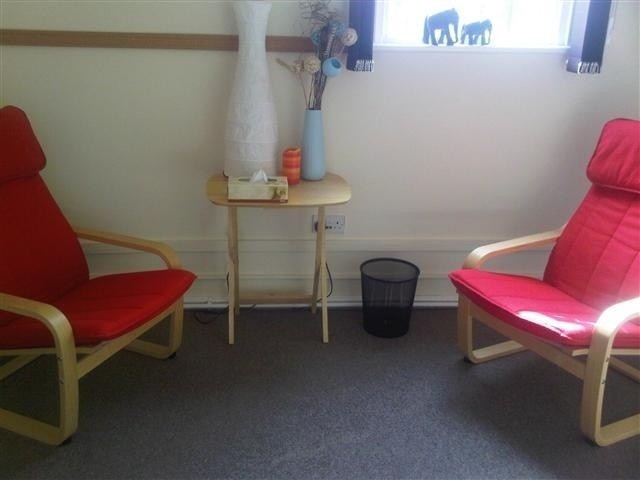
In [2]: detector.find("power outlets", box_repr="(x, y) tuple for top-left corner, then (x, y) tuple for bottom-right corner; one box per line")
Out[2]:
(311, 215), (345, 235)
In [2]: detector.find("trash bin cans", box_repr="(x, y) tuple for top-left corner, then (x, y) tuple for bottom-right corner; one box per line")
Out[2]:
(360, 258), (420, 338)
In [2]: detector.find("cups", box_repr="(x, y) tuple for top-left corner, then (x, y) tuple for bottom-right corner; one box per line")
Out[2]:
(283, 145), (301, 184)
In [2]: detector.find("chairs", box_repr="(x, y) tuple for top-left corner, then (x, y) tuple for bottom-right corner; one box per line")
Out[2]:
(449, 117), (640, 447)
(0, 106), (196, 446)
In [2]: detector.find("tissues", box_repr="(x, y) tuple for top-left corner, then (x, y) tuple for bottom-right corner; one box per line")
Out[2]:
(226, 169), (289, 203)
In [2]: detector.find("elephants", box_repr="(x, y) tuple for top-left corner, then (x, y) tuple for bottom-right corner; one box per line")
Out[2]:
(460, 19), (493, 45)
(423, 8), (459, 46)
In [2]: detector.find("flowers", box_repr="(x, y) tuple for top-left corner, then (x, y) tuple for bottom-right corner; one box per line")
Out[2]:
(276, 0), (357, 110)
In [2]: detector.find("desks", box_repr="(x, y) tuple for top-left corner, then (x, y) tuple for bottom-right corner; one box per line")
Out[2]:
(206, 172), (351, 344)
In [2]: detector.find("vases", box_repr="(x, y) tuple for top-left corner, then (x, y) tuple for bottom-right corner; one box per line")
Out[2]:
(302, 110), (326, 181)
